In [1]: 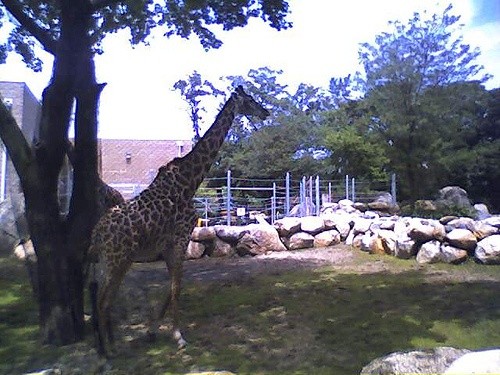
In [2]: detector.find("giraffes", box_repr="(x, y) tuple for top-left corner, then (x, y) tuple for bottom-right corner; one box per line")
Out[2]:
(85, 84), (271, 359)
(97, 80), (126, 219)
